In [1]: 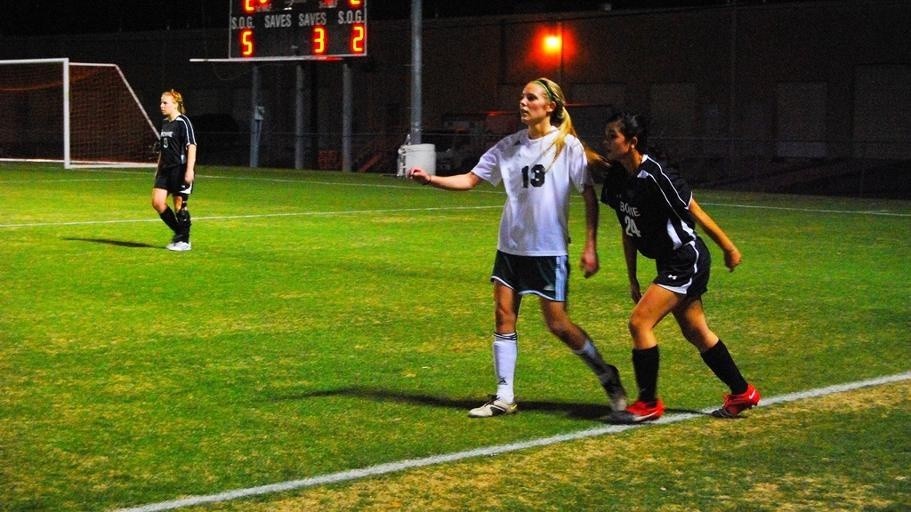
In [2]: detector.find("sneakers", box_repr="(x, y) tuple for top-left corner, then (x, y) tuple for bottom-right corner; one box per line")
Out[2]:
(609, 397), (664, 423)
(713, 385), (760, 417)
(468, 395), (519, 417)
(167, 242), (191, 252)
(601, 365), (626, 410)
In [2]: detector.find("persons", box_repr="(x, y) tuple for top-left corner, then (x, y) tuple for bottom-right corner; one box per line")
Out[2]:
(149, 89), (198, 252)
(402, 75), (628, 423)
(599, 108), (761, 422)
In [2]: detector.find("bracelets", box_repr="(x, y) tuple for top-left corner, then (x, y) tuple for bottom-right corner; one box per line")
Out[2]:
(422, 175), (432, 186)
